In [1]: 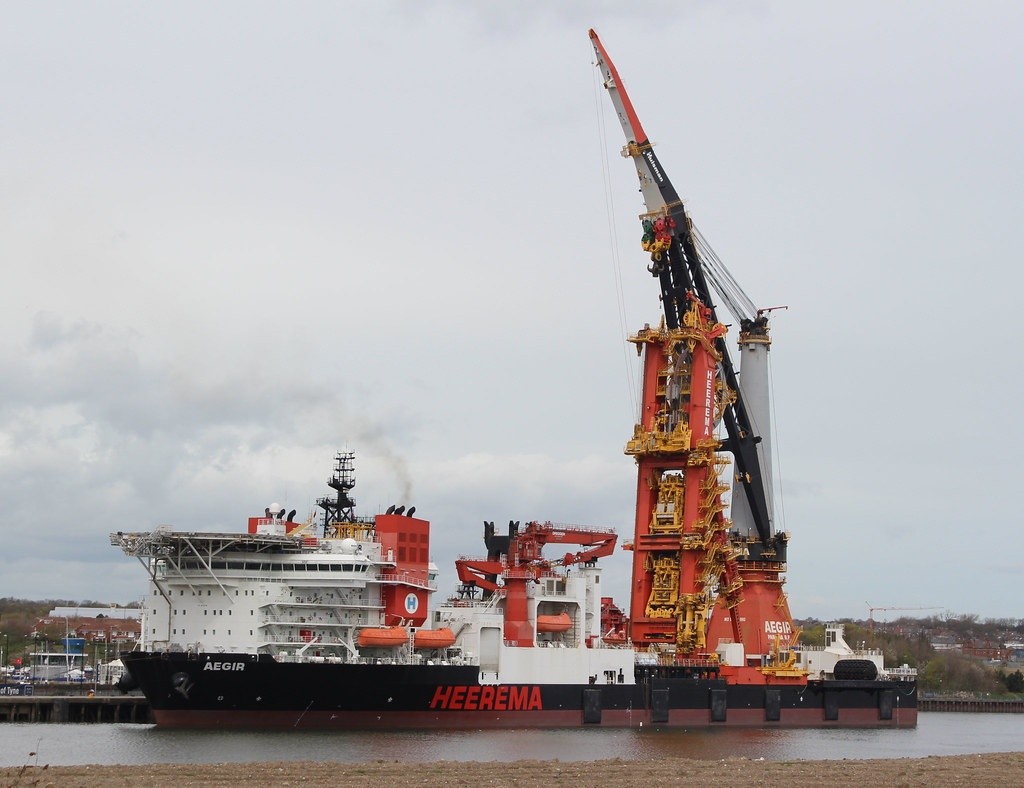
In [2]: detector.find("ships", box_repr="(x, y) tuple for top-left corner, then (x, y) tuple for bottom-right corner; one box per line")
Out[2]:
(105, 28), (923, 734)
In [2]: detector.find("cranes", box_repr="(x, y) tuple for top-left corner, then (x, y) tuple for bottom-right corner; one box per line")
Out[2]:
(867, 606), (942, 637)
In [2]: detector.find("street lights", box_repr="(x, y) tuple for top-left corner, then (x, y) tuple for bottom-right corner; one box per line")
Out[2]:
(3, 634), (8, 684)
(24, 635), (28, 671)
(33, 632), (38, 681)
